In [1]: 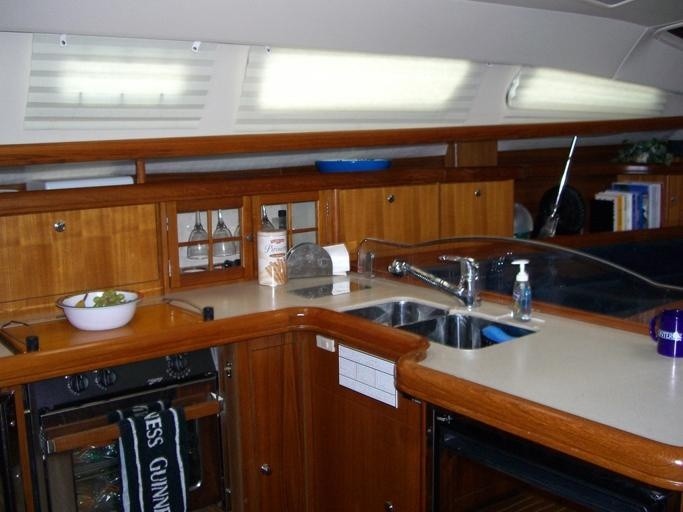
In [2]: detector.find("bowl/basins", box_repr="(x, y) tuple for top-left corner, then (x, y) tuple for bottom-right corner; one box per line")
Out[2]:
(55, 289), (145, 331)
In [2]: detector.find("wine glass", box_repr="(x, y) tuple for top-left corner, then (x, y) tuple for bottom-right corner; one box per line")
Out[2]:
(187, 205), (274, 260)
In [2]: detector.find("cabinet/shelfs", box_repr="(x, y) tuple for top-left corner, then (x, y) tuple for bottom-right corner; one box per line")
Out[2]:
(223, 328), (427, 511)
(166, 168), (325, 292)
(324, 169), (439, 257)
(2, 175), (165, 323)
(439, 169), (513, 242)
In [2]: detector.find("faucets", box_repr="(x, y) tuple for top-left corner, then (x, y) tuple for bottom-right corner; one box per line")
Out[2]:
(387, 253), (483, 310)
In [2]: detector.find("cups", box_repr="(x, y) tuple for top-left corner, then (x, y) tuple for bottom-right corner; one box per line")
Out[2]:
(649, 309), (683, 358)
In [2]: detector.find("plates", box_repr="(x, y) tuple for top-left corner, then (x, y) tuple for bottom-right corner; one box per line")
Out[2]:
(511, 184), (585, 239)
(315, 159), (390, 172)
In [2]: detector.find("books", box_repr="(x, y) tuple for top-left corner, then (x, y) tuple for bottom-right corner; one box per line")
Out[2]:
(594, 179), (662, 232)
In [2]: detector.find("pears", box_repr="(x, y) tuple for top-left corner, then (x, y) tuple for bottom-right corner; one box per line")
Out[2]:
(75, 294), (88, 308)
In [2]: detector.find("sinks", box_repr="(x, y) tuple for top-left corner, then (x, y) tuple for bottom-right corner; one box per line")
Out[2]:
(393, 310), (539, 350)
(333, 295), (453, 327)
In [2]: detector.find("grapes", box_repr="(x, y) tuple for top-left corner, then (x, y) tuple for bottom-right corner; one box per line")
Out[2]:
(94, 289), (125, 307)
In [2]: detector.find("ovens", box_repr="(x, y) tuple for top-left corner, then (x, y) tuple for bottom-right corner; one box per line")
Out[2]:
(22, 346), (230, 512)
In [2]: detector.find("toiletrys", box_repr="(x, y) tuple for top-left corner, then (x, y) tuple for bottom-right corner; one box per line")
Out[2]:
(512, 258), (532, 321)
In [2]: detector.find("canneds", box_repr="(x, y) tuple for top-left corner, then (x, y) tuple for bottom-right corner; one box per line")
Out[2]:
(256, 230), (289, 287)
